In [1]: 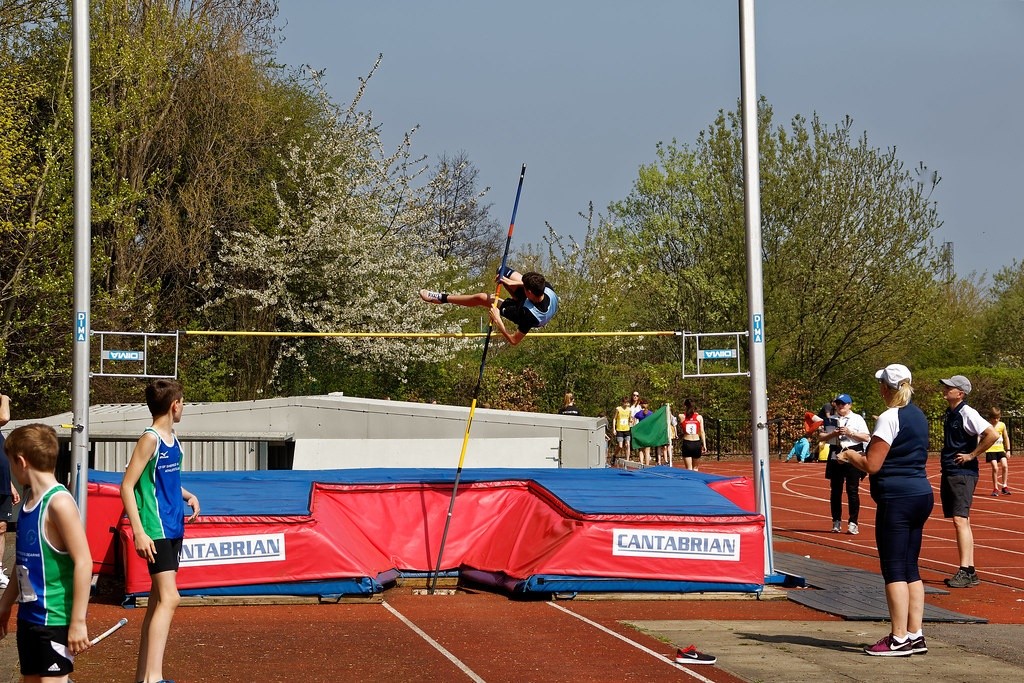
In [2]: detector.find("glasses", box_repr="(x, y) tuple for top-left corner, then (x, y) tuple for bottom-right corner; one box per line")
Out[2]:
(878, 378), (886, 384)
(836, 402), (847, 406)
(633, 395), (639, 397)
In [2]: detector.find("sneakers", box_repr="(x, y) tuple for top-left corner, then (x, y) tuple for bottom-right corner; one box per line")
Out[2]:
(1002, 488), (1011, 495)
(675, 645), (717, 664)
(847, 521), (860, 534)
(911, 636), (928, 653)
(970, 571), (980, 585)
(864, 633), (913, 656)
(831, 521), (841, 532)
(944, 570), (973, 587)
(419, 289), (443, 304)
(992, 491), (999, 496)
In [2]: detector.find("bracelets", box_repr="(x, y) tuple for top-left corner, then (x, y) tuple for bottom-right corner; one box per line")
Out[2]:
(1006, 450), (1010, 451)
(850, 431), (855, 438)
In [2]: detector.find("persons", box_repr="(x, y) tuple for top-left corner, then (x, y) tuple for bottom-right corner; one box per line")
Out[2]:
(629, 389), (642, 459)
(558, 392), (579, 416)
(818, 393), (871, 535)
(816, 397), (838, 431)
(661, 402), (679, 464)
(677, 399), (707, 471)
(819, 440), (831, 463)
(418, 265), (559, 346)
(634, 401), (654, 466)
(610, 395), (632, 466)
(939, 375), (1000, 588)
(121, 379), (200, 683)
(985, 406), (1012, 496)
(0, 392), (20, 589)
(782, 434), (819, 464)
(835, 364), (934, 656)
(0, 423), (93, 683)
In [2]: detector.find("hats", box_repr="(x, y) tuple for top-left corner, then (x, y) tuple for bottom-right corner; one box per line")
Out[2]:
(940, 375), (972, 395)
(875, 364), (912, 389)
(833, 394), (852, 404)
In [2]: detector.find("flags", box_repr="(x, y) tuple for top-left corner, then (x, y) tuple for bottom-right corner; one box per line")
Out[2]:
(803, 411), (824, 433)
(631, 405), (670, 450)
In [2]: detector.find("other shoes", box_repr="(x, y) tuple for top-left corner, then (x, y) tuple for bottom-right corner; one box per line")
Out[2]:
(0, 568), (10, 588)
